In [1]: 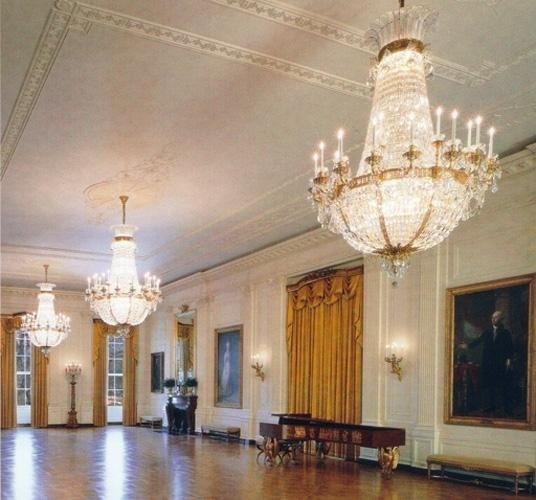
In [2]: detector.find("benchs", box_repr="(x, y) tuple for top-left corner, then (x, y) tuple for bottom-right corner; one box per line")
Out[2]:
(202, 424), (240, 442)
(425, 455), (535, 496)
(139, 415), (162, 429)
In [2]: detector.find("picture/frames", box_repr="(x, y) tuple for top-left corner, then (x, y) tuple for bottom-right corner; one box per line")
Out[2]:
(150, 351), (164, 394)
(213, 324), (243, 410)
(443, 272), (536, 431)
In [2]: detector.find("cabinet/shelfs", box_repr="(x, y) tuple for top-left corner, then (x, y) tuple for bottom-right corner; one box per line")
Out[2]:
(166, 395), (199, 436)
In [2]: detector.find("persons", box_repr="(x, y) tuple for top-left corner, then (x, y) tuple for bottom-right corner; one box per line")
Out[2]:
(459, 310), (514, 417)
(218, 341), (232, 390)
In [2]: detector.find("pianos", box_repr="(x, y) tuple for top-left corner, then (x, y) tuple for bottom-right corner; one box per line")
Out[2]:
(257, 413), (406, 479)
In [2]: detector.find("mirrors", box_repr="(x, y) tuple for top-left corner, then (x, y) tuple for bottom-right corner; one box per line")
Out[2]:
(174, 304), (197, 397)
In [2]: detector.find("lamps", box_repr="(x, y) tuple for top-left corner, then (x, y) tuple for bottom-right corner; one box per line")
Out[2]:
(306, 0), (503, 291)
(64, 360), (81, 428)
(250, 354), (264, 382)
(383, 341), (403, 382)
(84, 195), (163, 337)
(19, 264), (71, 360)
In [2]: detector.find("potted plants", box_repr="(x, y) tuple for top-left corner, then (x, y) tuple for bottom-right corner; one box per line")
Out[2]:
(163, 377), (198, 396)
